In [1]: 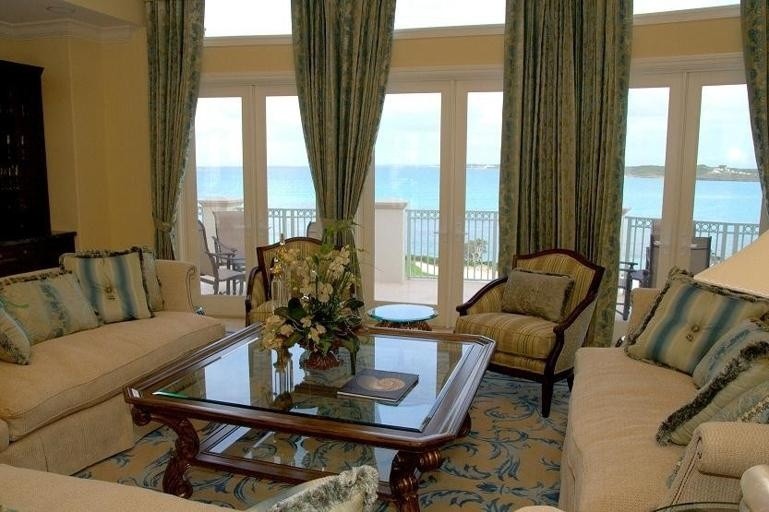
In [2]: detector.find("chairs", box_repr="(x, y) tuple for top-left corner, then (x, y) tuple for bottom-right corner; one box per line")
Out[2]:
(616, 235), (712, 321)
(305, 220), (325, 238)
(211, 207), (249, 271)
(192, 219), (246, 299)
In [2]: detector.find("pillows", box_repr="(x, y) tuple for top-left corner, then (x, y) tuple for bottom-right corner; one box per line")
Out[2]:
(0, 269), (106, 345)
(0, 297), (31, 365)
(651, 341), (769, 446)
(620, 281), (661, 342)
(623, 267), (769, 378)
(139, 244), (164, 311)
(502, 265), (575, 325)
(234, 462), (387, 512)
(59, 246), (152, 323)
(690, 318), (769, 390)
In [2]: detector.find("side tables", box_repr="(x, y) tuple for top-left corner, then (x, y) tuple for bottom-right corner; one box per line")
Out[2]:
(367, 304), (438, 330)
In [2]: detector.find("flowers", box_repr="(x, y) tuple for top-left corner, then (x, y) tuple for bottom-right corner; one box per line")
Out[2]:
(254, 240), (376, 372)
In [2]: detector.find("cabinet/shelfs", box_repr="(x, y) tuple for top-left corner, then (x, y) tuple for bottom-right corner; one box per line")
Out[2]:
(0, 227), (79, 280)
(0, 60), (51, 241)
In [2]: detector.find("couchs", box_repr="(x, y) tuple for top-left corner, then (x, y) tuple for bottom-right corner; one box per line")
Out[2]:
(0, 257), (224, 477)
(557, 286), (769, 512)
(455, 247), (606, 419)
(245, 235), (363, 330)
(0, 457), (574, 512)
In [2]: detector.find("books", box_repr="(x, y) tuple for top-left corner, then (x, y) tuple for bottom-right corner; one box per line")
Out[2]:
(337, 368), (420, 402)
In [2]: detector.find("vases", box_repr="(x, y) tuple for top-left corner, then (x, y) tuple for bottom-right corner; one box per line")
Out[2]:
(298, 339), (341, 369)
